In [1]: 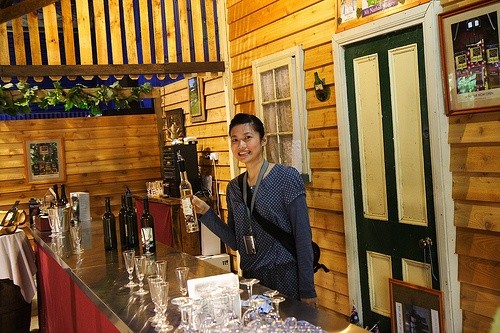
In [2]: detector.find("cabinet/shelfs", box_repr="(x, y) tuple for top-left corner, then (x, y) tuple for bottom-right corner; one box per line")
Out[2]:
(133, 192), (218, 256)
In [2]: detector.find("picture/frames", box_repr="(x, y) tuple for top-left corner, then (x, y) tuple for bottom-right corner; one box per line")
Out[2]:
(188, 76), (205, 123)
(165, 108), (185, 138)
(437, 0), (500, 117)
(389, 278), (445, 333)
(334, 0), (432, 34)
(21, 135), (66, 186)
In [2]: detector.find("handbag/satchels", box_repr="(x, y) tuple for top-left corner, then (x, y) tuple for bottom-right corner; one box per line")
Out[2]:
(290, 235), (321, 268)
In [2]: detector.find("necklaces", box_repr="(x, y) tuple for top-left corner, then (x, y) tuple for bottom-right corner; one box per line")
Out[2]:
(246, 171), (258, 190)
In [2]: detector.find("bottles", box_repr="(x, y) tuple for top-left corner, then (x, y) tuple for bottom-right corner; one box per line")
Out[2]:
(178, 159), (199, 234)
(1, 199), (20, 226)
(140, 197), (156, 256)
(105, 250), (119, 303)
(103, 197), (117, 250)
(119, 194), (139, 251)
(53, 183), (68, 209)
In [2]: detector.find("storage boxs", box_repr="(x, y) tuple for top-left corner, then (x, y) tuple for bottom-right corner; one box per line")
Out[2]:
(69, 191), (90, 221)
(197, 253), (230, 272)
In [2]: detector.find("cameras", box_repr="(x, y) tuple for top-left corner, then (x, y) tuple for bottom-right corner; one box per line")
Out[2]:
(242, 235), (257, 255)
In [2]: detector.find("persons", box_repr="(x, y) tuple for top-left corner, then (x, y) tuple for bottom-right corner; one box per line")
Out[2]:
(180, 113), (318, 311)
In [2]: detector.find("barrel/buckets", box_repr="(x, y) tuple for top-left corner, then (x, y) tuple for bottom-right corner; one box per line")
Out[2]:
(47, 206), (70, 231)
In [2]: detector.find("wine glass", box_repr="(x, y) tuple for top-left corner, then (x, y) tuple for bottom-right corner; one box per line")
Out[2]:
(123, 250), (324, 333)
(146, 180), (164, 198)
